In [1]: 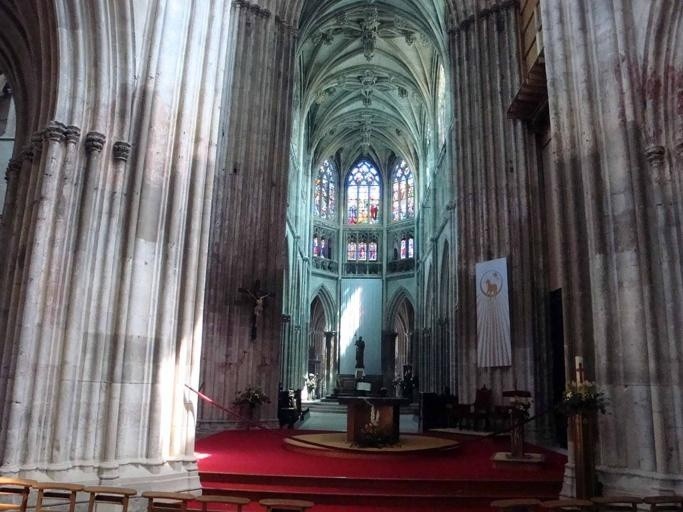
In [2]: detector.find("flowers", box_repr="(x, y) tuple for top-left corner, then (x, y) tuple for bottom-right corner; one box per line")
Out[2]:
(230, 382), (272, 405)
(347, 416), (403, 449)
(560, 378), (611, 416)
(506, 393), (535, 419)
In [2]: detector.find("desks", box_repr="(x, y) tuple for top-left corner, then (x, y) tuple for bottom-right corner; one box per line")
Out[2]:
(336, 394), (410, 443)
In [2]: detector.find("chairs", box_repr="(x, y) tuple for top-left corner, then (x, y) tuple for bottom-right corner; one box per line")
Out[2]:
(489, 487), (683, 511)
(459, 382), (493, 432)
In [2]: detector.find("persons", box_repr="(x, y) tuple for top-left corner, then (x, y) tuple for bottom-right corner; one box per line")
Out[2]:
(245, 288), (271, 332)
(354, 336), (365, 369)
